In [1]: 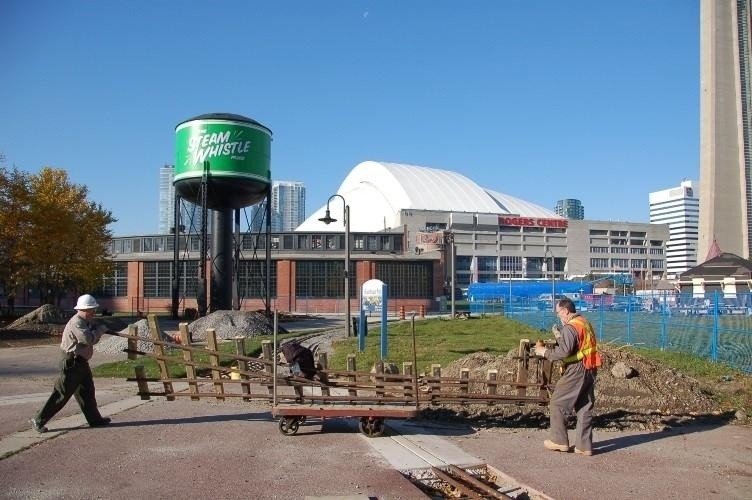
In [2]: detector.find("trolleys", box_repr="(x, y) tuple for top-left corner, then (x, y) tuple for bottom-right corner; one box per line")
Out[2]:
(268, 306), (422, 439)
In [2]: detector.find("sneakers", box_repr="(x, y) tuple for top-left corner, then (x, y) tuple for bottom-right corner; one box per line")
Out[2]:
(89, 416), (111, 426)
(543, 439), (570, 452)
(574, 447), (593, 456)
(27, 415), (48, 433)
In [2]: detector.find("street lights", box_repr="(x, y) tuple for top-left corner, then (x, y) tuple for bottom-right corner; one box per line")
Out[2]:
(543, 247), (557, 323)
(509, 268), (516, 319)
(316, 192), (353, 337)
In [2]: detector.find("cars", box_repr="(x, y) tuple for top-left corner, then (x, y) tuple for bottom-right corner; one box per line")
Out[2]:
(538, 293), (752, 316)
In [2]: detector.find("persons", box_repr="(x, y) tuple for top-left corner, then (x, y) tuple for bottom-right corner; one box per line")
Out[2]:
(533, 298), (604, 458)
(28, 294), (112, 435)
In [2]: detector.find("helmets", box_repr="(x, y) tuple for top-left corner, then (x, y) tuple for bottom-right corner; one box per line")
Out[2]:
(73, 294), (99, 310)
